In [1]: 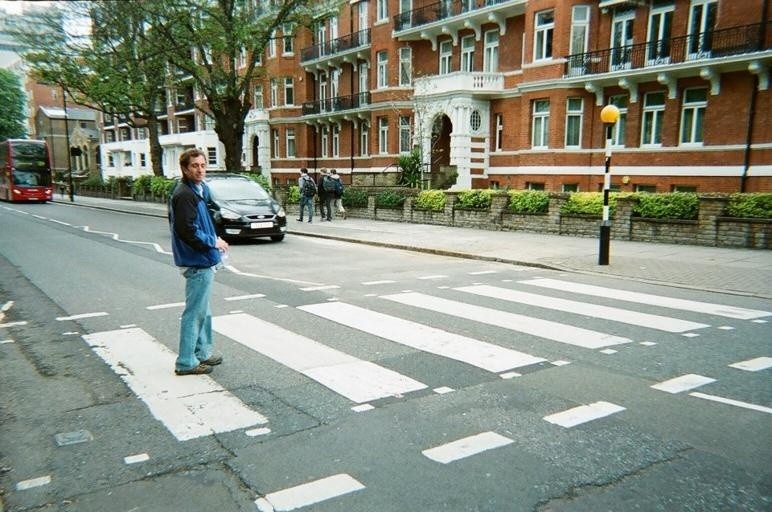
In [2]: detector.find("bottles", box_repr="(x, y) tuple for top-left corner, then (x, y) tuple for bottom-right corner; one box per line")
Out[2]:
(219, 248), (231, 268)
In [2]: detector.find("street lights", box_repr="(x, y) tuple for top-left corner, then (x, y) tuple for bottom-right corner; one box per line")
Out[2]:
(598, 103), (622, 267)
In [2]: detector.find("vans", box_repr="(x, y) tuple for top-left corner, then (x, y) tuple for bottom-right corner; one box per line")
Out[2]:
(165, 171), (288, 245)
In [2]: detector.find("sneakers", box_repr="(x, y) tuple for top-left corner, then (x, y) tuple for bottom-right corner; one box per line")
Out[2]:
(321, 212), (346, 220)
(176, 355), (222, 375)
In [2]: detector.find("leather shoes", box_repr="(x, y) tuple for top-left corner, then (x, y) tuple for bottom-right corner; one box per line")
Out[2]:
(297, 218), (312, 222)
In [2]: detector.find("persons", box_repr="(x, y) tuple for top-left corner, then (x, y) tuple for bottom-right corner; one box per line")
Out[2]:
(296, 168), (318, 223)
(168, 149), (229, 375)
(318, 168), (332, 221)
(329, 169), (347, 220)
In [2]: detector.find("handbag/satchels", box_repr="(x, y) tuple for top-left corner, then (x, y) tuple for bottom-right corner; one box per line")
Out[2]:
(208, 200), (224, 237)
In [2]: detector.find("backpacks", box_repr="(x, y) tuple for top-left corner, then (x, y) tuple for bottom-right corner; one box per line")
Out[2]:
(303, 177), (315, 196)
(322, 175), (343, 194)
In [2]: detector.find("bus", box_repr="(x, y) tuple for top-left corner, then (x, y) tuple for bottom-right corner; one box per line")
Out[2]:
(0, 138), (54, 204)
